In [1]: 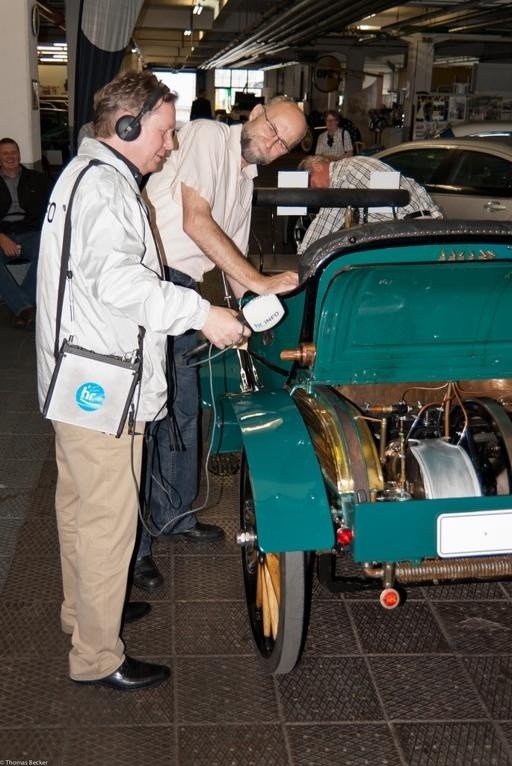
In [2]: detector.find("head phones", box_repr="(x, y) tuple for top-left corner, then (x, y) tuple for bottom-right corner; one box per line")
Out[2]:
(116, 82), (169, 141)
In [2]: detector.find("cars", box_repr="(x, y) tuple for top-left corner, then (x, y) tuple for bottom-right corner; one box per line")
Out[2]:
(352, 121), (512, 220)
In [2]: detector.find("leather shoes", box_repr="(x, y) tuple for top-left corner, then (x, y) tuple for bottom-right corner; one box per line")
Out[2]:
(175, 523), (226, 543)
(129, 554), (165, 592)
(70, 653), (171, 689)
(120, 601), (152, 625)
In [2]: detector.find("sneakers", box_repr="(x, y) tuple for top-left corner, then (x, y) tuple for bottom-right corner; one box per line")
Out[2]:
(11, 312), (36, 329)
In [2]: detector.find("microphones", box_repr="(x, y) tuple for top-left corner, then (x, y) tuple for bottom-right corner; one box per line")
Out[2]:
(182, 293), (286, 362)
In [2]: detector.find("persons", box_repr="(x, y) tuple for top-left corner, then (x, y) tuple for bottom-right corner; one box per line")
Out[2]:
(0, 137), (55, 328)
(131, 95), (308, 594)
(315, 110), (353, 158)
(77, 93), (95, 148)
(34, 71), (254, 692)
(190, 89), (213, 120)
(295, 154), (443, 258)
(339, 108), (405, 141)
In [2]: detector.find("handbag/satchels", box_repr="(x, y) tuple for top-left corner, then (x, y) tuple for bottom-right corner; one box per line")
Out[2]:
(42, 337), (141, 439)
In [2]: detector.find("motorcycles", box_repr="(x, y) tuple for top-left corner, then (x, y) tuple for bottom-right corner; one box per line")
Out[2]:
(182, 188), (512, 676)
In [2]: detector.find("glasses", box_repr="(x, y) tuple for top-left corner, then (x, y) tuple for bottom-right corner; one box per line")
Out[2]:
(256, 107), (291, 154)
